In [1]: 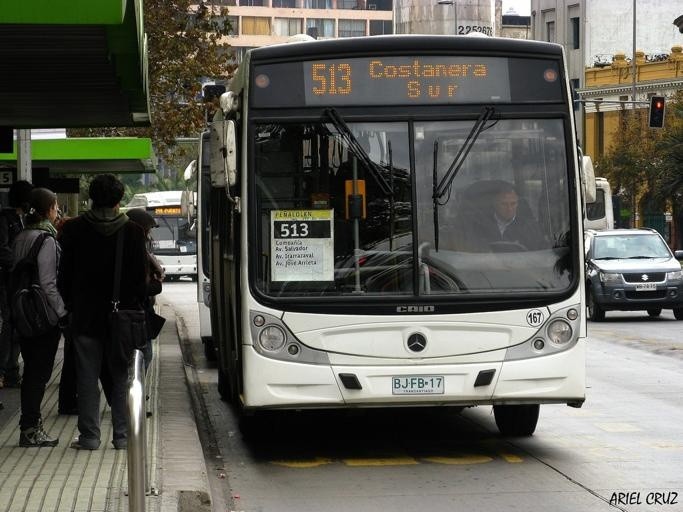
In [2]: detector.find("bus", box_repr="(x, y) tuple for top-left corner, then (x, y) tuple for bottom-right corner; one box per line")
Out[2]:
(210, 33), (588, 446)
(438, 135), (516, 223)
(184, 131), (212, 364)
(127, 189), (196, 282)
(582, 177), (613, 257)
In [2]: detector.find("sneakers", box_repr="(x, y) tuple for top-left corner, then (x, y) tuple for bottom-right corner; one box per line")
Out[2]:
(71, 441), (91, 449)
(19, 413), (59, 447)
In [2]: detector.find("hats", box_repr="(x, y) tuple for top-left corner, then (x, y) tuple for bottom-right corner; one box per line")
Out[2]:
(88, 174), (124, 209)
(125, 209), (158, 232)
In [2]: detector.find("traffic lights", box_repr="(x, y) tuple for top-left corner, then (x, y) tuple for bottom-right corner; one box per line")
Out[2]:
(647, 95), (665, 129)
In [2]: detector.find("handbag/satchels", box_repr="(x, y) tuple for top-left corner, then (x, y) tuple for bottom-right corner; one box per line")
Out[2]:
(109, 309), (149, 350)
(14, 232), (59, 337)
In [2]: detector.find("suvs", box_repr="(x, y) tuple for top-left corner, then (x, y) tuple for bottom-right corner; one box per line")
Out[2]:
(586, 225), (683, 321)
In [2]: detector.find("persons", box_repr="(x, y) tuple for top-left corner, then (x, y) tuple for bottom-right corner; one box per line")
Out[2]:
(6, 186), (62, 452)
(0, 172), (158, 414)
(459, 185), (529, 255)
(55, 173), (146, 453)
(335, 137), (376, 180)
